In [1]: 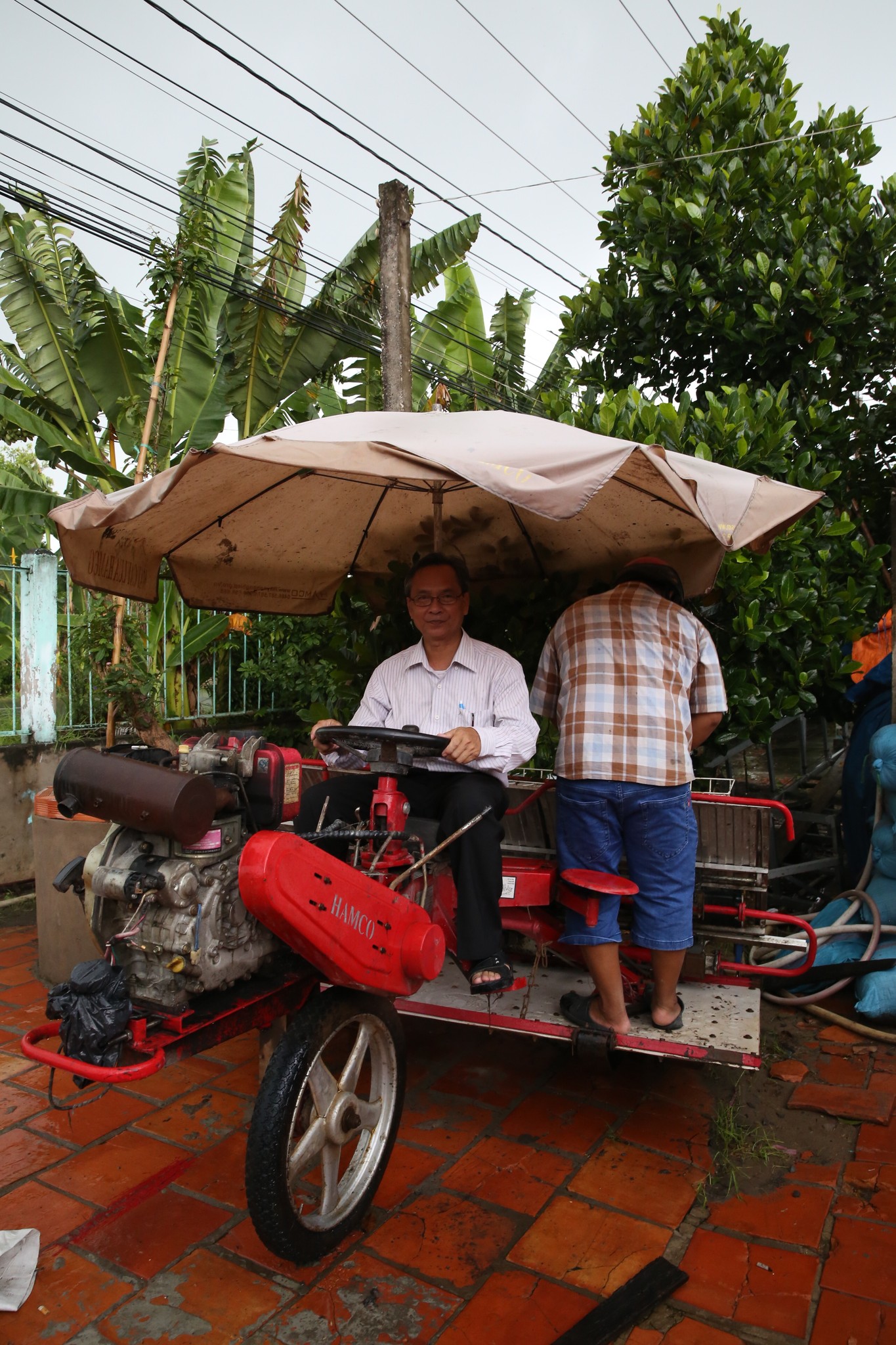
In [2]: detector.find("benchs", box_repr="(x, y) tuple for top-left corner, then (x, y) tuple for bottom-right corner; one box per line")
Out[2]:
(502, 785), (772, 892)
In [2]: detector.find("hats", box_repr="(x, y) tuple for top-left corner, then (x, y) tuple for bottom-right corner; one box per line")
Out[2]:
(617, 556), (684, 606)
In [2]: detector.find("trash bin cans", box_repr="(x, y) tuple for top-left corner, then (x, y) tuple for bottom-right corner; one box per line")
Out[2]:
(32, 785), (112, 983)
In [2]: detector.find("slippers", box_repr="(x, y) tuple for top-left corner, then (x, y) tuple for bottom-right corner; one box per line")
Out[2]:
(647, 992), (684, 1030)
(560, 992), (631, 1034)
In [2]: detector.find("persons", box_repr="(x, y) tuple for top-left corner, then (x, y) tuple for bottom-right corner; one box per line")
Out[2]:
(529, 559), (728, 1035)
(294, 552), (540, 994)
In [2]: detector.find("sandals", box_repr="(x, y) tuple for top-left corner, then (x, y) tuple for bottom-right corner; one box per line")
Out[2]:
(469, 953), (514, 994)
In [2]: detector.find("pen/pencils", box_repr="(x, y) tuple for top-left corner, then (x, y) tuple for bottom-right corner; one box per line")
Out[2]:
(471, 712), (475, 727)
(459, 701), (464, 709)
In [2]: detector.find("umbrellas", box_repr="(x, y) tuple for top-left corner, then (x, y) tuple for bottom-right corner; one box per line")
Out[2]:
(48, 390), (828, 622)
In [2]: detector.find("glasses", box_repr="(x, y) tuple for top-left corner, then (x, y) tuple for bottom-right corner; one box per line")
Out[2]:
(411, 591), (461, 607)
